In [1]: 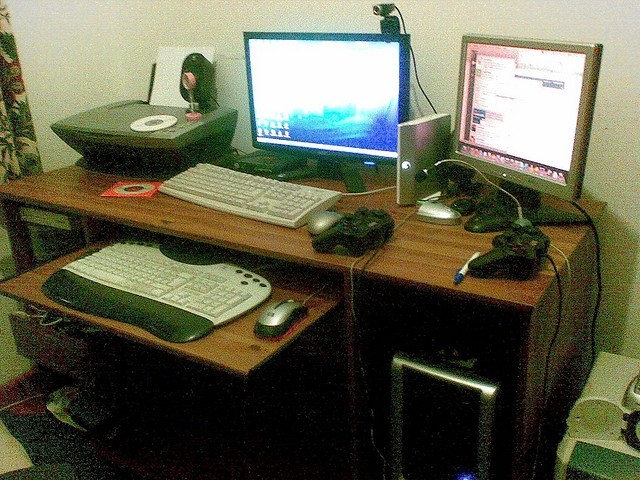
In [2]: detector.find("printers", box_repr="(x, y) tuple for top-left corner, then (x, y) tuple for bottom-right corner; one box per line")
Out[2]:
(51, 45), (237, 177)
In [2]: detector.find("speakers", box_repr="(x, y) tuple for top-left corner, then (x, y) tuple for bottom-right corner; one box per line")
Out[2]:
(180, 52), (214, 114)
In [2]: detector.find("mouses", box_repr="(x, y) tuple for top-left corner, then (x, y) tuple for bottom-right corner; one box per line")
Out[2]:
(308, 209), (343, 234)
(253, 296), (307, 337)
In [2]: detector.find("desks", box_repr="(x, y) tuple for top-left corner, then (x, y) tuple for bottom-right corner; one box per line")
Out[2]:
(1, 158), (608, 480)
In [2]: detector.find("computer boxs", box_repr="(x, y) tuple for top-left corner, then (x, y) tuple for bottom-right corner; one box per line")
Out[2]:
(391, 345), (498, 476)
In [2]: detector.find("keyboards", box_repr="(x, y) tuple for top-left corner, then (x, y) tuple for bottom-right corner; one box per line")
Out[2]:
(40, 237), (273, 344)
(158, 162), (345, 228)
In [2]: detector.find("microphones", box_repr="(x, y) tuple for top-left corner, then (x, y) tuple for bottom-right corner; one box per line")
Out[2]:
(414, 157), (532, 228)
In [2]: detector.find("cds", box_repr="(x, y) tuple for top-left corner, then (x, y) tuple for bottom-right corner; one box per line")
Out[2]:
(113, 183), (154, 195)
(130, 114), (182, 132)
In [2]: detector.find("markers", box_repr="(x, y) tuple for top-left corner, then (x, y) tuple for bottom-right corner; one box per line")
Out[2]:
(453, 252), (481, 284)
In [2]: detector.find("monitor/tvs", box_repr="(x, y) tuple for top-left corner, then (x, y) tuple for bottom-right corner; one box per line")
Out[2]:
(445, 33), (605, 234)
(242, 32), (410, 191)
